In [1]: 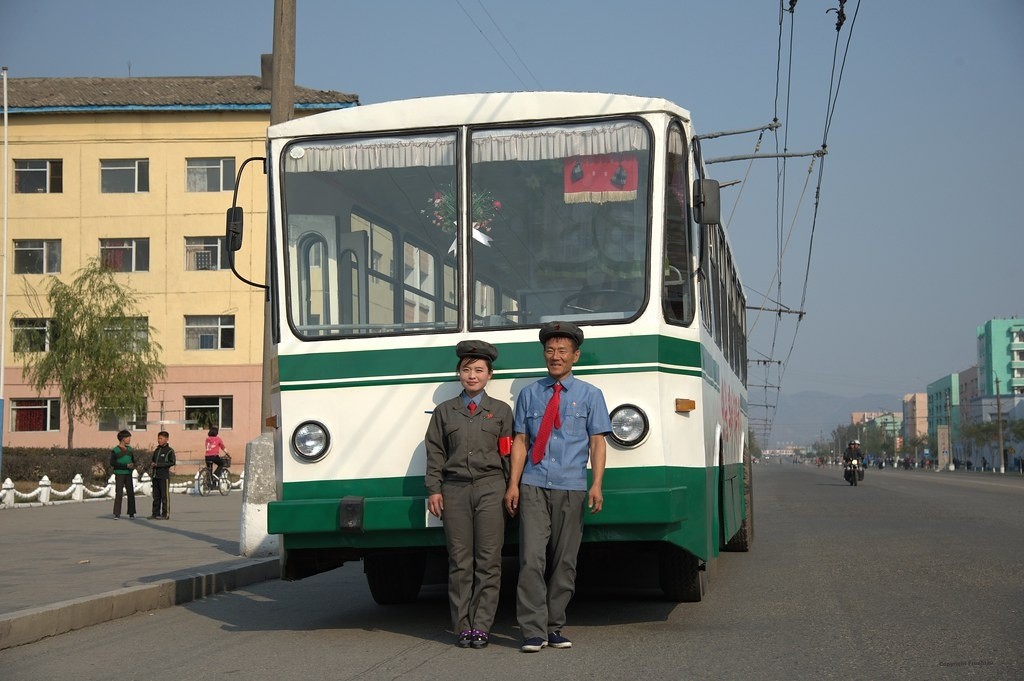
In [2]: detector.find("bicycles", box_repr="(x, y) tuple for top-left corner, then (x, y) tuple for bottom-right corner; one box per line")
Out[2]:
(197, 452), (232, 496)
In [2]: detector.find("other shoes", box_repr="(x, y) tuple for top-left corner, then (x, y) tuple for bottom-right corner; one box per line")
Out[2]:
(213, 473), (220, 481)
(148, 515), (169, 520)
(457, 629), (488, 648)
(114, 514), (135, 520)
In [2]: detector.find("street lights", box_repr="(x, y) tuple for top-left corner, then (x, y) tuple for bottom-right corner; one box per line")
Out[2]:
(971, 364), (1006, 473)
(925, 386), (956, 471)
(801, 407), (897, 468)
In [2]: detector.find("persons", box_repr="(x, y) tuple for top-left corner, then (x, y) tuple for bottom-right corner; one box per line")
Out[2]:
(110, 430), (136, 519)
(426, 340), (515, 647)
(817, 441), (986, 481)
(205, 427), (230, 489)
(506, 321), (614, 653)
(148, 432), (176, 519)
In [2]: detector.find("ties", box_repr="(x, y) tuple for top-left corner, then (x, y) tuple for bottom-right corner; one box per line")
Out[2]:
(531, 385), (563, 465)
(467, 403), (477, 415)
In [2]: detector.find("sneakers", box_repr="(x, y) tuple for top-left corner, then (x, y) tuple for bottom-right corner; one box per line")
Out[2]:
(522, 632), (571, 651)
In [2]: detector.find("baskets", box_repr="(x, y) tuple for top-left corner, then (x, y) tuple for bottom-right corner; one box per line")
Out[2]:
(220, 456), (231, 468)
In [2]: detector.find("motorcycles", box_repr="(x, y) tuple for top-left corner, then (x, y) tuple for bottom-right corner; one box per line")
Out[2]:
(844, 453), (865, 486)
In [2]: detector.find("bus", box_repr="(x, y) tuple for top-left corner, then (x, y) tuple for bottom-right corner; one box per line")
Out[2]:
(223, 90), (756, 600)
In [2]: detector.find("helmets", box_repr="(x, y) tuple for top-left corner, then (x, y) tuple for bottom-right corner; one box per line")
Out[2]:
(848, 440), (861, 445)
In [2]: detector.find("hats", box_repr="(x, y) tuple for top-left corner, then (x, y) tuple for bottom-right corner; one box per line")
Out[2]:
(456, 340), (497, 361)
(539, 322), (584, 345)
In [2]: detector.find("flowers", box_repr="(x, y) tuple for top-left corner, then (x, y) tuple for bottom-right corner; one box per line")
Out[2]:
(418, 172), (506, 234)
